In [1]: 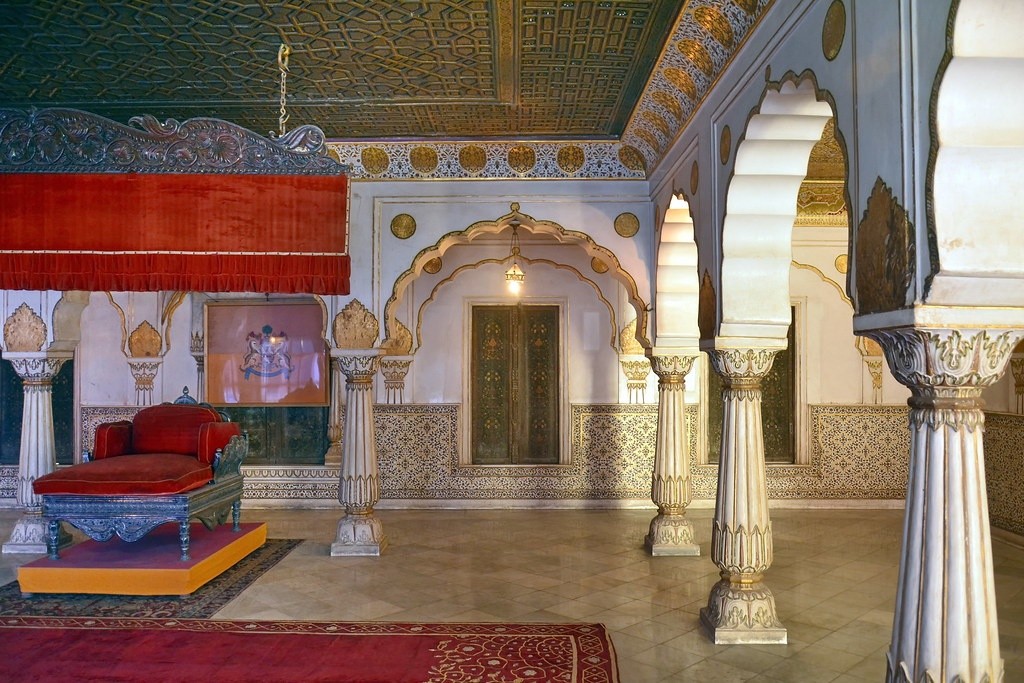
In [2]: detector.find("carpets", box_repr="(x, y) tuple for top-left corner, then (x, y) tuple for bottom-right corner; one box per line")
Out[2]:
(0, 620), (621, 682)
(0, 539), (306, 621)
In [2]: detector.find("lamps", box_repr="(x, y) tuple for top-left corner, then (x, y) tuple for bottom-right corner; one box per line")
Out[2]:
(505, 219), (525, 293)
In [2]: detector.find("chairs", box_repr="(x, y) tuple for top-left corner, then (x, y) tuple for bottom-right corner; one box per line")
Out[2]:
(32, 385), (248, 561)
